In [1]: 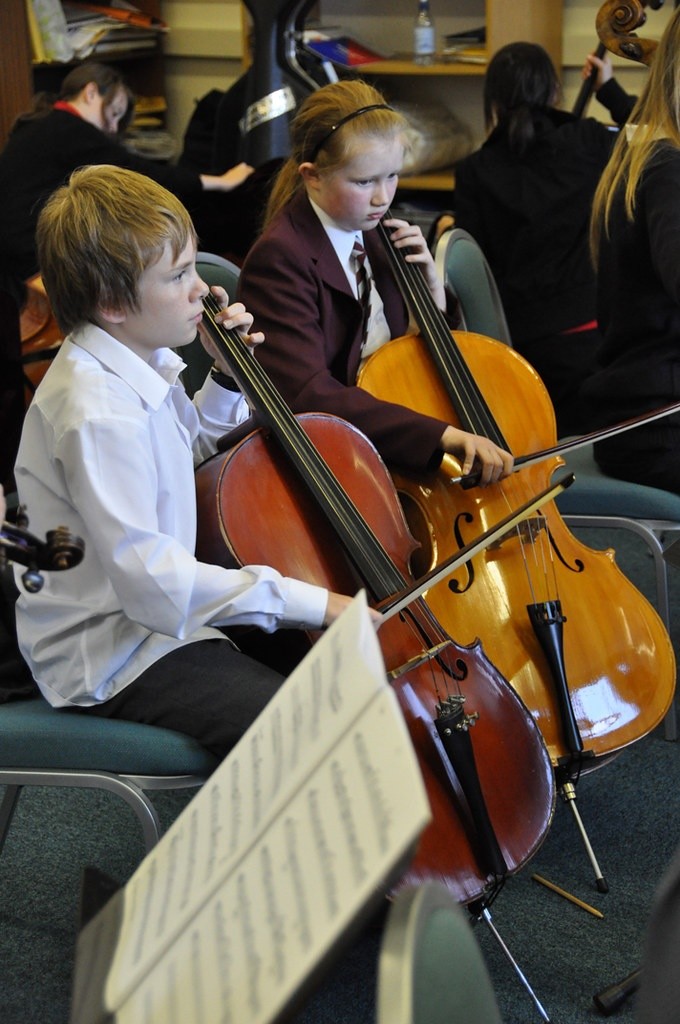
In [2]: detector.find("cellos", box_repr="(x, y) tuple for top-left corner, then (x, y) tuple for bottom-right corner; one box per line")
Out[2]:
(571, 0), (666, 119)
(357, 212), (678, 898)
(197, 293), (559, 1024)
(595, 0), (659, 67)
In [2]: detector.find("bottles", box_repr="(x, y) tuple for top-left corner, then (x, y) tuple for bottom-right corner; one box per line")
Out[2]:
(414, 2), (434, 65)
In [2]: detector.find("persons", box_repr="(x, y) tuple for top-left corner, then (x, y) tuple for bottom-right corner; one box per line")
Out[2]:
(14, 165), (407, 1024)
(1, 9), (679, 497)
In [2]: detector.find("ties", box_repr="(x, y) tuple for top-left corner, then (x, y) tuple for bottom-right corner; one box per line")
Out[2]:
(351, 236), (372, 373)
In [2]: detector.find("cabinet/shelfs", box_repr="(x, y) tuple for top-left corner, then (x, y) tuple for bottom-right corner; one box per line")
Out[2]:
(240, 0), (563, 189)
(0, 0), (165, 156)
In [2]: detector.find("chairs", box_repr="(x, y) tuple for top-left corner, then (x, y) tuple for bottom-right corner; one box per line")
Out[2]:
(436, 227), (680, 740)
(181, 252), (244, 402)
(0, 697), (213, 859)
